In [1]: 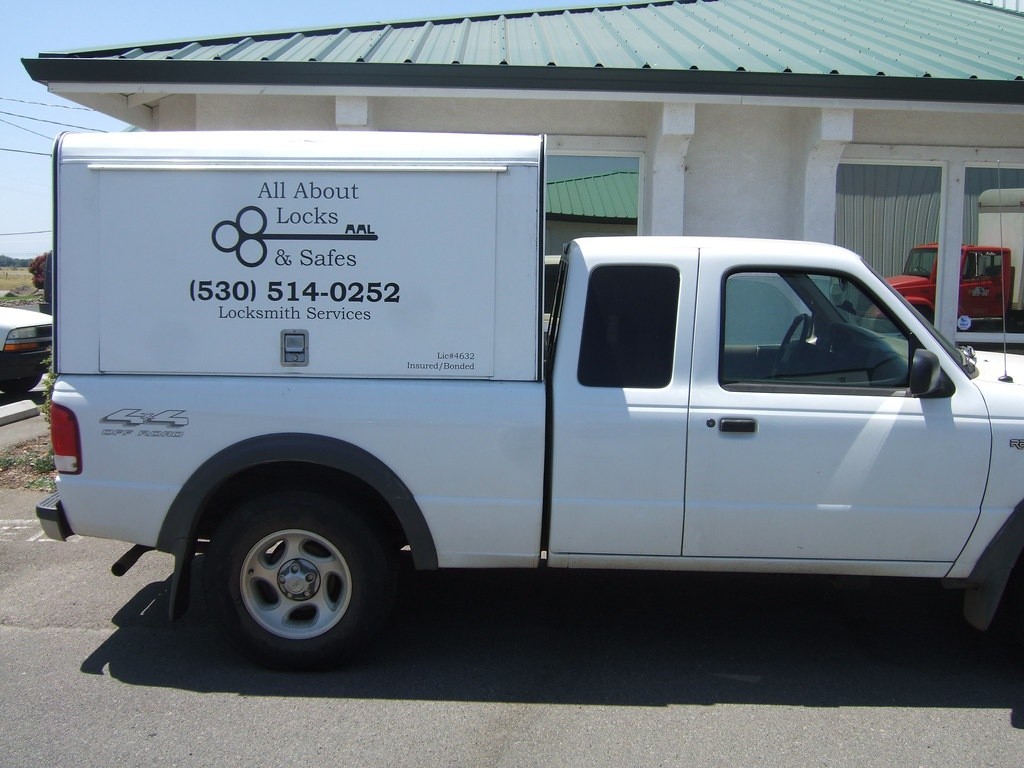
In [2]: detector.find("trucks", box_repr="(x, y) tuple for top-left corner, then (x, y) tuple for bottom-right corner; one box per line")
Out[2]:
(859, 188), (1023, 334)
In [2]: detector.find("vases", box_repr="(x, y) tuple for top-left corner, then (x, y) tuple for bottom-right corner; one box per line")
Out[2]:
(33, 281), (44, 289)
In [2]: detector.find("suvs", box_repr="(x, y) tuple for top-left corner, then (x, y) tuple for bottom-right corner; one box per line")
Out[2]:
(538, 255), (819, 353)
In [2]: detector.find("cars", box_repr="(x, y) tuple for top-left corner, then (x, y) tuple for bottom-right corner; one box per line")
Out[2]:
(0, 307), (52, 391)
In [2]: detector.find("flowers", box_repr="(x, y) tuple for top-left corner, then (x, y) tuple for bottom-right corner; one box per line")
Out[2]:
(29, 255), (46, 282)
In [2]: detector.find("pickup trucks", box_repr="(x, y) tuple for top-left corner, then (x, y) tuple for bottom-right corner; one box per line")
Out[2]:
(27, 128), (1023, 674)
